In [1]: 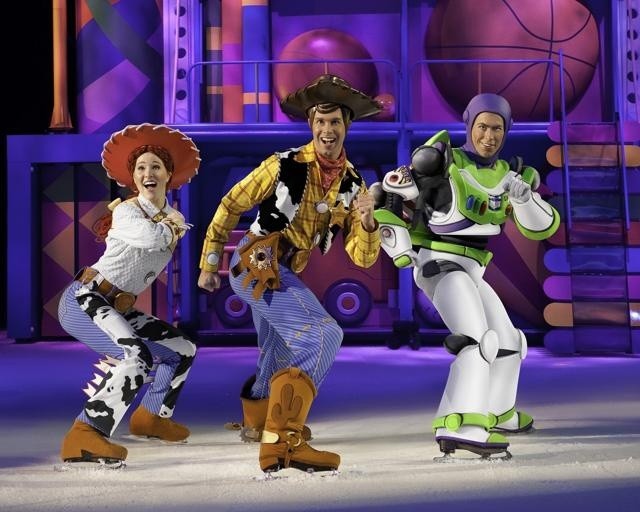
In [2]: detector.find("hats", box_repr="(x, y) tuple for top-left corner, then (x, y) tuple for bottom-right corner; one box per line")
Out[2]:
(100, 123), (201, 192)
(281, 74), (382, 121)
(463, 93), (513, 164)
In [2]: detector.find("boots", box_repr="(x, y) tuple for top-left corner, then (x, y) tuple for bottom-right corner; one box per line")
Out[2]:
(240, 374), (311, 442)
(259, 367), (340, 472)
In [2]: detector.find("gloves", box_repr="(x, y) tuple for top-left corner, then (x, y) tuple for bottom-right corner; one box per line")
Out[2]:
(503, 177), (531, 204)
(393, 250), (421, 269)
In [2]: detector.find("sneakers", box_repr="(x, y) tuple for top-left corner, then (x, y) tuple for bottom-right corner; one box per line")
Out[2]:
(435, 425), (511, 454)
(487, 412), (534, 432)
(129, 406), (189, 441)
(60, 420), (128, 460)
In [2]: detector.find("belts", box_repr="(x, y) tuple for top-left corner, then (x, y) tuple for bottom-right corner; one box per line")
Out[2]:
(76, 267), (135, 312)
(278, 240), (311, 275)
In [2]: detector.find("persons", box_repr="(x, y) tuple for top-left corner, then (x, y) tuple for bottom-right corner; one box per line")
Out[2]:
(197, 75), (382, 472)
(58, 123), (203, 463)
(369, 93), (561, 453)
